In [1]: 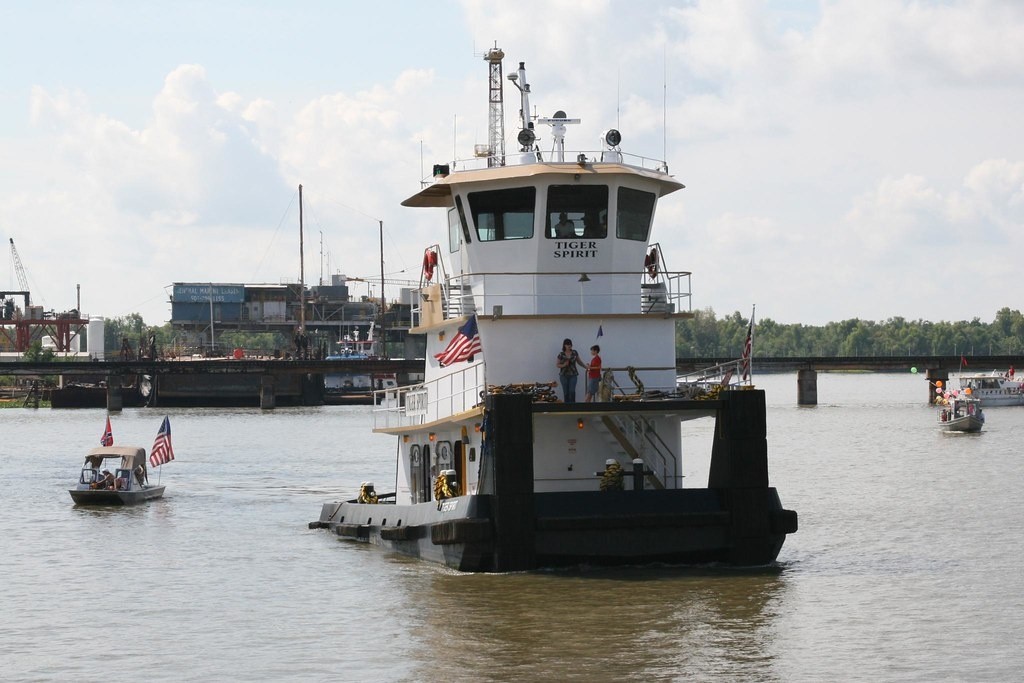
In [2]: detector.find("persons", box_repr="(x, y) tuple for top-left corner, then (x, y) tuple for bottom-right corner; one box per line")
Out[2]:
(556, 338), (588, 402)
(980, 409), (985, 423)
(293, 329), (308, 360)
(89, 466), (114, 490)
(941, 409), (947, 422)
(968, 365), (1015, 393)
(956, 401), (975, 415)
(554, 209), (575, 239)
(585, 345), (601, 402)
(571, 214), (606, 239)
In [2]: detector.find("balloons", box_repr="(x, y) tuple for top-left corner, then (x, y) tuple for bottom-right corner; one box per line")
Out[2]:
(965, 388), (971, 394)
(936, 381), (942, 387)
(952, 390), (958, 397)
(910, 367), (917, 374)
(936, 388), (949, 405)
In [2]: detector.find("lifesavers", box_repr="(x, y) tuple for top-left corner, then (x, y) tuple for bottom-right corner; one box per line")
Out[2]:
(423, 249), (434, 281)
(361, 484), (377, 503)
(648, 248), (659, 278)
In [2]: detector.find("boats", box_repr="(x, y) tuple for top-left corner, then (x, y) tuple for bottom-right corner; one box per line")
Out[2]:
(325, 321), (399, 390)
(306, 39), (801, 584)
(935, 369), (1024, 434)
(68, 444), (168, 507)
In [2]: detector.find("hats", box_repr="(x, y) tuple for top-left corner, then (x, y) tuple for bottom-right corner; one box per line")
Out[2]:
(102, 468), (109, 473)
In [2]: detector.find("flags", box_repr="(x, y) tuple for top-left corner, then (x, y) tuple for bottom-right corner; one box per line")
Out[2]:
(433, 314), (483, 367)
(149, 415), (175, 468)
(961, 354), (968, 366)
(100, 416), (113, 447)
(740, 321), (752, 380)
